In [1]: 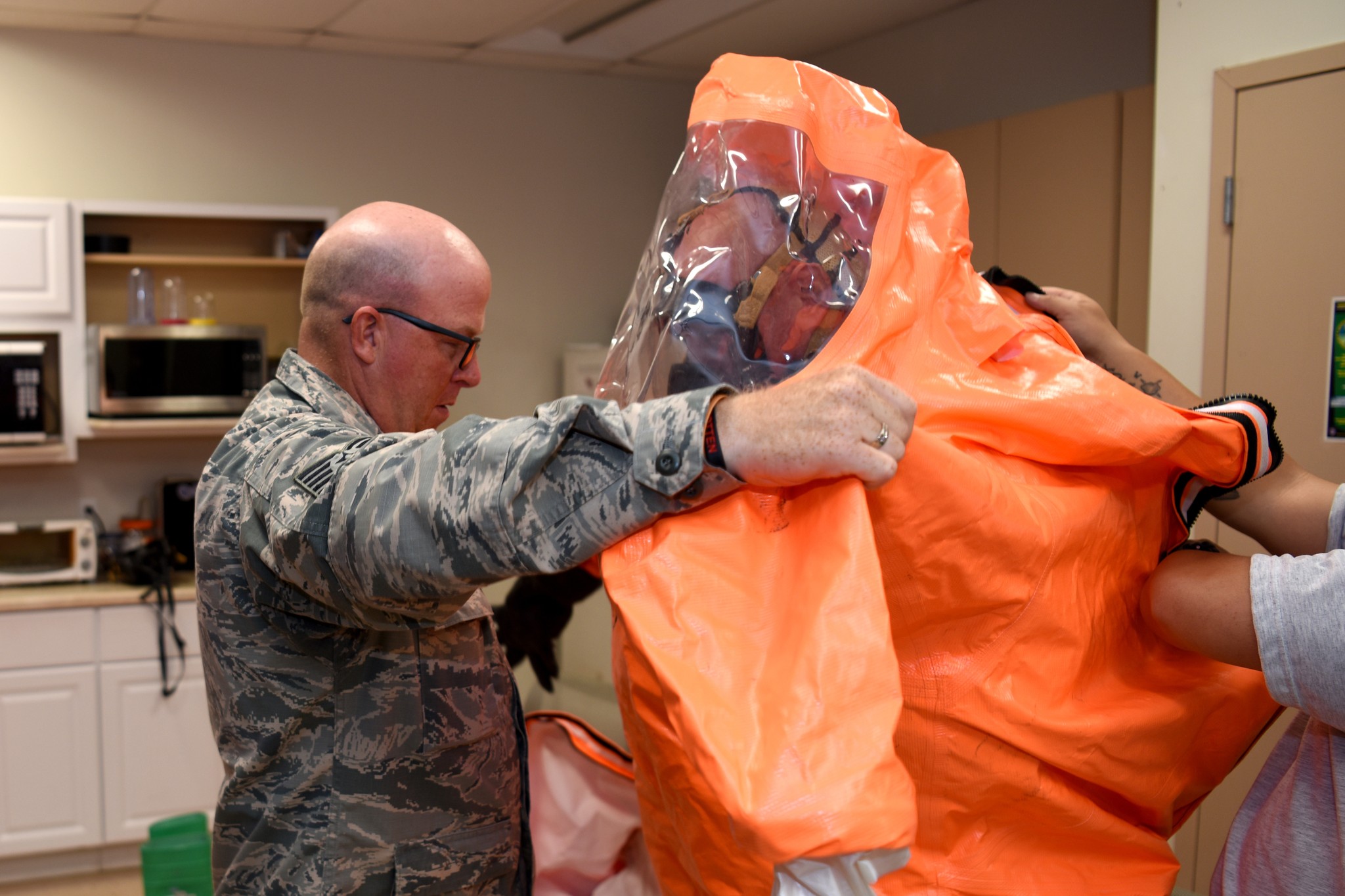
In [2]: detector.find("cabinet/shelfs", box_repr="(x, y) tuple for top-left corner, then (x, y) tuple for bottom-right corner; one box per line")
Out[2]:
(0, 198), (337, 886)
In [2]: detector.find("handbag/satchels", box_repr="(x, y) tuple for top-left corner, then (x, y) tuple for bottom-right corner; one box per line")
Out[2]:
(117, 536), (184, 697)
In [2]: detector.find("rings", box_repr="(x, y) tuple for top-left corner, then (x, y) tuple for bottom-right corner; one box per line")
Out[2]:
(871, 422), (890, 450)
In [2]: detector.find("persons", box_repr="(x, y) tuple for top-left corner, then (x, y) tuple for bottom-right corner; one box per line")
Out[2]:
(1023, 286), (1345, 896)
(191, 197), (918, 896)
(497, 55), (978, 896)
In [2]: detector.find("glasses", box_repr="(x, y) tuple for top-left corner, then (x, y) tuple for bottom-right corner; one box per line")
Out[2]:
(341, 307), (481, 370)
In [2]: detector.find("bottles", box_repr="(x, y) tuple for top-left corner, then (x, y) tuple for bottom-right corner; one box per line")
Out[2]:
(126, 267), (156, 325)
(120, 518), (155, 554)
(165, 276), (184, 322)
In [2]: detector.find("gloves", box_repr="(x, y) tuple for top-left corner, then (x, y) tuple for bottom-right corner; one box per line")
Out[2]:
(490, 562), (603, 693)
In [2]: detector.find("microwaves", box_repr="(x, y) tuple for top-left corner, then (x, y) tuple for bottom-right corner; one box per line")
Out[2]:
(0, 340), (46, 444)
(87, 322), (268, 415)
(0, 519), (99, 585)
